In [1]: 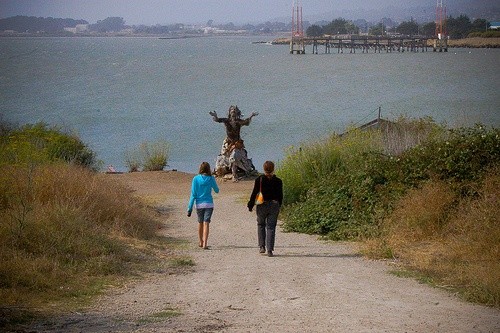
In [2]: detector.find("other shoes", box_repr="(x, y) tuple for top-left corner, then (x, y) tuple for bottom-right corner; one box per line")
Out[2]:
(199, 240), (203, 246)
(260, 246), (266, 253)
(268, 250), (273, 257)
(203, 244), (207, 248)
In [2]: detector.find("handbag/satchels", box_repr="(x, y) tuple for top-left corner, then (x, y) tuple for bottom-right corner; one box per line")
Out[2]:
(254, 176), (264, 205)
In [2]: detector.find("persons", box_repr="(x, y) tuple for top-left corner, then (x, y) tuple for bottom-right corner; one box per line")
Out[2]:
(247, 161), (283, 256)
(187, 162), (219, 250)
(209, 105), (259, 182)
